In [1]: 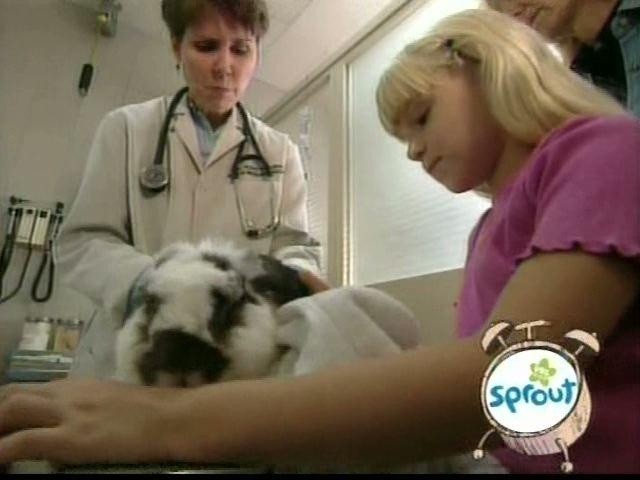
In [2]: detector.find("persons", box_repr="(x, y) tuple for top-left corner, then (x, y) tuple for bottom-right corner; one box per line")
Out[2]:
(2, 1), (639, 478)
(484, 0), (640, 122)
(52, 1), (332, 381)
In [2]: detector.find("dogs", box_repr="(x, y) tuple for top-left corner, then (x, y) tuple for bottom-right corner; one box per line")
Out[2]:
(115, 230), (309, 386)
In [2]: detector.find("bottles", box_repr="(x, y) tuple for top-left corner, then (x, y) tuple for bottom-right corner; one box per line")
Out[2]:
(55, 318), (83, 354)
(21, 316), (53, 352)
